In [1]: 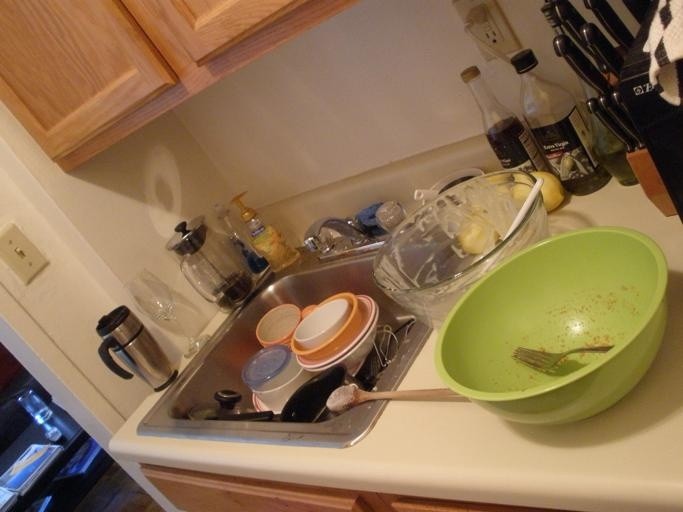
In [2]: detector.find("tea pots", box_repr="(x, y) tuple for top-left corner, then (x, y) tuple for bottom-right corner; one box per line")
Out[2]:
(165, 215), (258, 310)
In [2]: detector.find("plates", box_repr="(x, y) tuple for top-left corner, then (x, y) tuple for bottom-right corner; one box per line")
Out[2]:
(253, 392), (281, 415)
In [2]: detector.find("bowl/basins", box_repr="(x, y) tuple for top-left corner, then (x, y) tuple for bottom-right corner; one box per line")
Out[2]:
(291, 292), (363, 362)
(240, 346), (299, 391)
(372, 168), (551, 336)
(256, 304), (301, 345)
(422, 168), (486, 205)
(295, 295), (375, 368)
(252, 369), (312, 411)
(433, 225), (668, 428)
(305, 304), (379, 372)
(294, 299), (350, 348)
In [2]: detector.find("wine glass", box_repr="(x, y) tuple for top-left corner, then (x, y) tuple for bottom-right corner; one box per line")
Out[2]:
(123, 268), (210, 357)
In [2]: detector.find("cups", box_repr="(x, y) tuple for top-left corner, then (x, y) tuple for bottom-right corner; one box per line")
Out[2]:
(95, 305), (178, 391)
(17, 388), (53, 425)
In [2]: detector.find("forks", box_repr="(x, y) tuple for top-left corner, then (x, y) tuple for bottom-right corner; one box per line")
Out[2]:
(511, 345), (613, 371)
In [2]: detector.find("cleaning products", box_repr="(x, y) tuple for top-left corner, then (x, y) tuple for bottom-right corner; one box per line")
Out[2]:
(227, 189), (303, 273)
(205, 200), (271, 275)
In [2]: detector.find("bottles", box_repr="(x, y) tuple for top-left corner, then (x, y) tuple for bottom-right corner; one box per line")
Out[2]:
(461, 66), (552, 172)
(578, 75), (639, 186)
(510, 48), (611, 196)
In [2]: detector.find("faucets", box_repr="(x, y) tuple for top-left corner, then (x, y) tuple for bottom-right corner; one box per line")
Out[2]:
(303, 214), (366, 255)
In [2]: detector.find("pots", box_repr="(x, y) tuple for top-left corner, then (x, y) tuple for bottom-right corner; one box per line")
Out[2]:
(279, 364), (366, 422)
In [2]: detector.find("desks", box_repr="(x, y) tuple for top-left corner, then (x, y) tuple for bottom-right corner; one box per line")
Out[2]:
(1, 400), (110, 511)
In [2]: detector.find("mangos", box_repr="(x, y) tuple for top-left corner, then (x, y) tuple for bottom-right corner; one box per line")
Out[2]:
(458, 170), (567, 253)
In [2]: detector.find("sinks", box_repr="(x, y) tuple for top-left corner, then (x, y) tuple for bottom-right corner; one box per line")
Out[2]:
(136, 249), (435, 449)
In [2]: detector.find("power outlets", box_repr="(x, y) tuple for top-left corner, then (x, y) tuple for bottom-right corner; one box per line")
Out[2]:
(453, 0), (522, 66)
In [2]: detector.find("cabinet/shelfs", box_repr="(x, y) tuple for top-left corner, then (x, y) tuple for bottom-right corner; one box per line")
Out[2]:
(0, 0), (356, 175)
(136, 464), (562, 511)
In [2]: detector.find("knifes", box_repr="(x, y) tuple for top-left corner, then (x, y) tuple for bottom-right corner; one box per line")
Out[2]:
(556, 48), (611, 75)
(613, 93), (639, 152)
(580, 77), (610, 134)
(550, 1), (649, 47)
